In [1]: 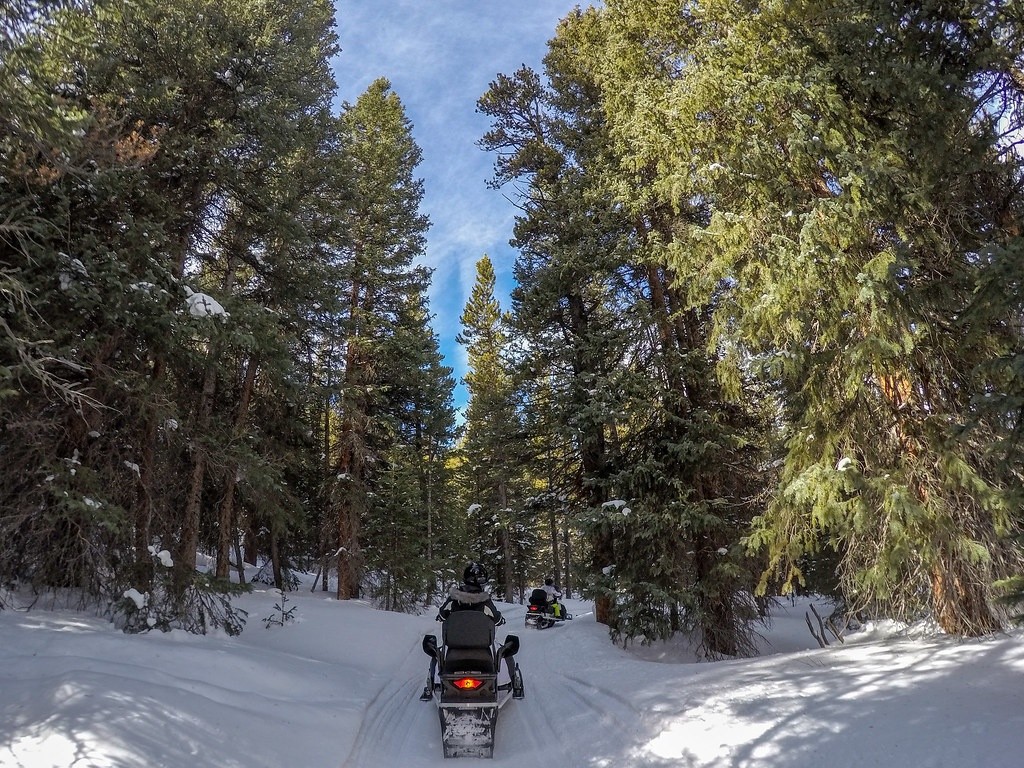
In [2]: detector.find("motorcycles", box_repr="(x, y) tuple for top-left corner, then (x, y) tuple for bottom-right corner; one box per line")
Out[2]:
(421, 609), (525, 758)
(523, 588), (572, 630)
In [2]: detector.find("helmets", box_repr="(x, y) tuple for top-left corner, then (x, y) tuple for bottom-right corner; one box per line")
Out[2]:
(464, 563), (488, 588)
(545, 578), (553, 585)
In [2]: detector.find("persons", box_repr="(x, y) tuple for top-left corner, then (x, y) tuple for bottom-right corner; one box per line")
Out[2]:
(541, 578), (566, 620)
(436, 563), (506, 627)
